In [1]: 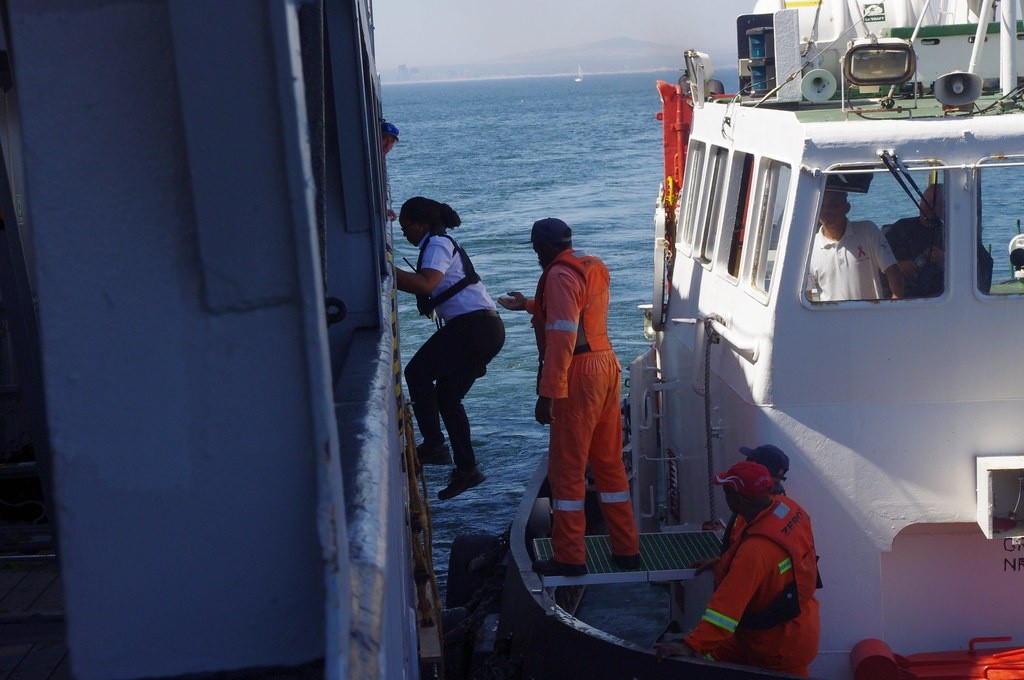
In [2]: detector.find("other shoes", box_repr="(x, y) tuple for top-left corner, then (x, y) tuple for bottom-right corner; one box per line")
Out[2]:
(533, 559), (585, 576)
(412, 441), (453, 466)
(438, 465), (487, 499)
(613, 552), (641, 571)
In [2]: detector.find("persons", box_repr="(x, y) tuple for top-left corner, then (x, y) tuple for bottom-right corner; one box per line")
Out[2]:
(498, 217), (642, 576)
(689, 444), (791, 593)
(805, 173), (906, 300)
(391, 196), (505, 500)
(886, 183), (952, 296)
(653, 461), (819, 679)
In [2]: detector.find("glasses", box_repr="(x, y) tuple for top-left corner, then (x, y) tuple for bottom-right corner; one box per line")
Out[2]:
(401, 220), (418, 231)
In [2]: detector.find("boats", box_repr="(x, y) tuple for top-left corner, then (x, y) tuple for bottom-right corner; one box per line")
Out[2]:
(442, 0), (1024, 680)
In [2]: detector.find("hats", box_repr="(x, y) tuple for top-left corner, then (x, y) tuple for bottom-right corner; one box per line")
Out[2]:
(714, 460), (771, 500)
(517, 218), (573, 245)
(382, 123), (400, 141)
(738, 444), (790, 478)
